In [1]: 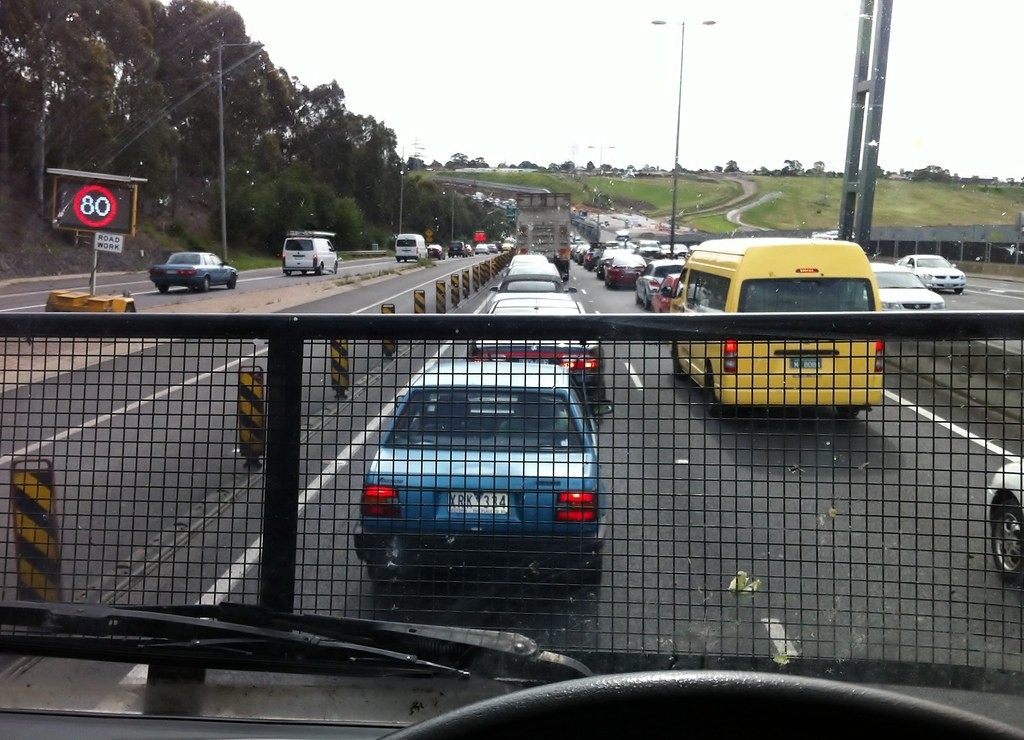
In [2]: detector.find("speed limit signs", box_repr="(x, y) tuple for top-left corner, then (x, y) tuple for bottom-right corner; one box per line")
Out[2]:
(74, 185), (117, 227)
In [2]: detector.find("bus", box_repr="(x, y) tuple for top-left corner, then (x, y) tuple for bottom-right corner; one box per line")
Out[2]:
(475, 244), (490, 255)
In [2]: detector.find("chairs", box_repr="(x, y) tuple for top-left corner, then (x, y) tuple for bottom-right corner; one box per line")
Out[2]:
(426, 395), (480, 435)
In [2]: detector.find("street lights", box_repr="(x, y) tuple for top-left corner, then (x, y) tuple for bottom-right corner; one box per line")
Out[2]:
(216, 40), (265, 261)
(587, 144), (616, 230)
(651, 18), (716, 259)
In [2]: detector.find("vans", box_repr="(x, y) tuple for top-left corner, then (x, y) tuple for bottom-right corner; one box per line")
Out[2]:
(661, 236), (884, 422)
(395, 234), (429, 262)
(282, 231), (339, 276)
(492, 241), (503, 253)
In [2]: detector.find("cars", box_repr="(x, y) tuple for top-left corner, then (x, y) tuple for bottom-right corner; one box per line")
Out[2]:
(985, 462), (1024, 584)
(466, 231), (699, 407)
(426, 244), (445, 260)
(148, 251), (239, 294)
(465, 245), (476, 257)
(862, 262), (946, 312)
(501, 243), (511, 251)
(896, 254), (966, 295)
(487, 243), (498, 253)
(471, 191), (517, 211)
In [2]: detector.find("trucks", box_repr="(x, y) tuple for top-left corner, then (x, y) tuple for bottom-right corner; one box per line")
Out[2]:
(515, 190), (571, 281)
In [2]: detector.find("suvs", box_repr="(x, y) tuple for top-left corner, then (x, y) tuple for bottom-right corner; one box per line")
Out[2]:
(448, 241), (468, 258)
(354, 359), (613, 601)
